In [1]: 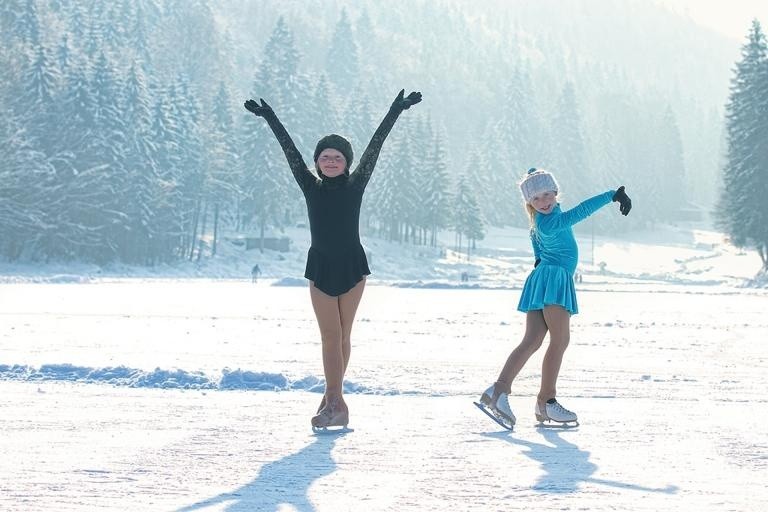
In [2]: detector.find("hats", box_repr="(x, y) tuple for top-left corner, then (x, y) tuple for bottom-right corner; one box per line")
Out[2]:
(521, 167), (558, 200)
(313, 134), (353, 165)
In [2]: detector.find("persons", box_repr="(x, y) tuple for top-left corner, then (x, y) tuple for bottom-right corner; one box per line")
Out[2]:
(241, 87), (423, 429)
(480, 167), (632, 424)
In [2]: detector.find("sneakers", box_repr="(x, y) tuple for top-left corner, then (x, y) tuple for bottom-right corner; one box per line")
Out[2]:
(481, 384), (515, 427)
(311, 394), (349, 427)
(534, 397), (577, 423)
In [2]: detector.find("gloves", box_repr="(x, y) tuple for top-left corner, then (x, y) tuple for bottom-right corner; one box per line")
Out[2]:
(393, 89), (422, 108)
(613, 185), (631, 216)
(244, 97), (272, 116)
